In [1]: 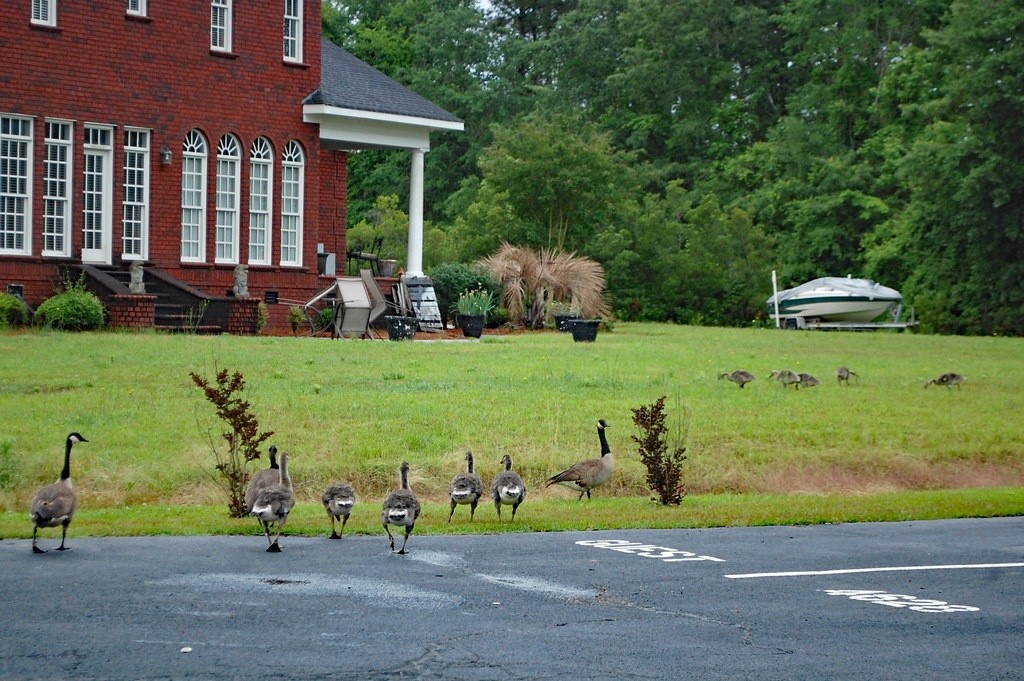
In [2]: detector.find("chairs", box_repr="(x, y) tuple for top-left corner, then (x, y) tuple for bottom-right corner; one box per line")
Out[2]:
(347, 236), (383, 276)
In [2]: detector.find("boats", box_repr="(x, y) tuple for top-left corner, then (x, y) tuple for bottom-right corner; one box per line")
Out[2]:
(767, 274), (902, 323)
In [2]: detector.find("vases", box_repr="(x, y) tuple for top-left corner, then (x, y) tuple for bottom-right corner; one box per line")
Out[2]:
(379, 259), (397, 276)
(567, 318), (601, 342)
(385, 315), (420, 341)
(458, 314), (485, 339)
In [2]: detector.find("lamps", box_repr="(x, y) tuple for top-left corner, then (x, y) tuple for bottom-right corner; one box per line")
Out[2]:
(160, 146), (173, 167)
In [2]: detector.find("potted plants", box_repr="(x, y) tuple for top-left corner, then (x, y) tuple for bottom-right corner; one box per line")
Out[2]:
(317, 309), (334, 333)
(288, 304), (306, 333)
(555, 302), (583, 331)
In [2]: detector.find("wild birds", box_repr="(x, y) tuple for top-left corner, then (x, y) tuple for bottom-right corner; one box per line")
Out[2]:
(449, 451), (483, 521)
(382, 462), (421, 555)
(245, 445), (296, 554)
(544, 419), (613, 502)
(322, 482), (354, 539)
(492, 454), (526, 521)
(718, 367), (860, 391)
(32, 432), (90, 553)
(925, 373), (967, 388)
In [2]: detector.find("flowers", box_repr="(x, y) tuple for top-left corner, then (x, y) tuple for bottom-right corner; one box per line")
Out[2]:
(448, 282), (495, 325)
